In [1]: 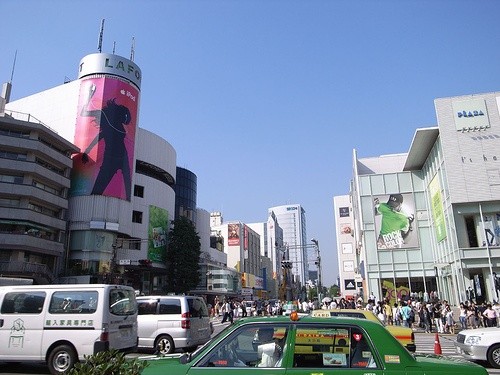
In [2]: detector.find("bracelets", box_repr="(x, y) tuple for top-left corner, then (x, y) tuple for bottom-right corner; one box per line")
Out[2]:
(409, 226), (414, 230)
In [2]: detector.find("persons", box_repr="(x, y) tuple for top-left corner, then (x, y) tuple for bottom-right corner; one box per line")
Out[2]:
(61, 296), (97, 313)
(480, 216), (494, 246)
(373, 194), (415, 249)
(492, 273), (500, 298)
(215, 290), (500, 368)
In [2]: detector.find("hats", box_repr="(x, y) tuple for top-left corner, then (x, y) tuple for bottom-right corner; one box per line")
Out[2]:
(387, 194), (403, 208)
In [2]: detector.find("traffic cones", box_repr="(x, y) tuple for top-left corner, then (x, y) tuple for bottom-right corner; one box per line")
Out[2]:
(434, 333), (441, 354)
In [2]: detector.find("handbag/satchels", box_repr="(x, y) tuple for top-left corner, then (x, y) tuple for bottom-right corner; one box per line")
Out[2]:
(407, 318), (413, 324)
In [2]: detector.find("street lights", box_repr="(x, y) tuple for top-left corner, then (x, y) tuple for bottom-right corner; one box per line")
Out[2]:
(310, 238), (320, 310)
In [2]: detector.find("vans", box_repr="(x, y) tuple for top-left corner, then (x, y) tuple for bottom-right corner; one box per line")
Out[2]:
(109, 296), (211, 354)
(0, 284), (139, 375)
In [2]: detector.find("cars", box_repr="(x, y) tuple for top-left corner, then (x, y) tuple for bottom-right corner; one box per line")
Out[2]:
(454, 326), (500, 369)
(252, 309), (416, 354)
(136, 313), (489, 375)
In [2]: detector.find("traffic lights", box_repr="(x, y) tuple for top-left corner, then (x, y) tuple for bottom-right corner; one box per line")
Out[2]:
(281, 261), (293, 270)
